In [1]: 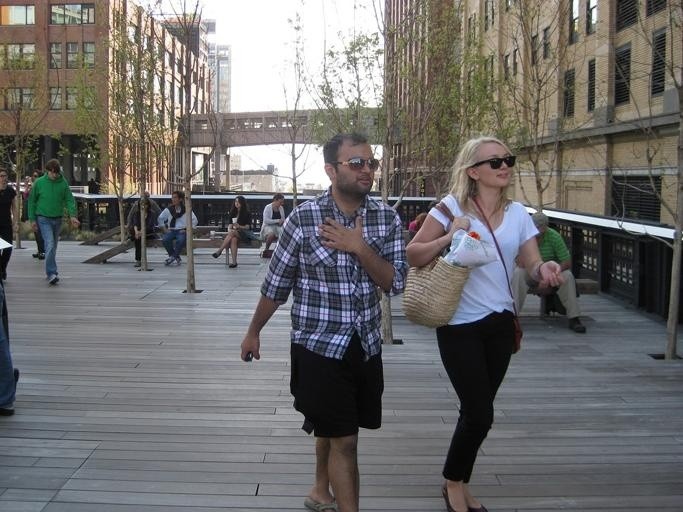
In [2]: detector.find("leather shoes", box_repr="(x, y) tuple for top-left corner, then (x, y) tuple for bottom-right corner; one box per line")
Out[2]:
(441, 480), (488, 512)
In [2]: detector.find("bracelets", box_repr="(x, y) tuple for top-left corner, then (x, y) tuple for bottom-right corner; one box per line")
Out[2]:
(536, 263), (543, 276)
(437, 239), (442, 250)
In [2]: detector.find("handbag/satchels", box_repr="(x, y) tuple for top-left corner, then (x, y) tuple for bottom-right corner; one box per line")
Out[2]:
(510, 318), (526, 352)
(403, 254), (470, 327)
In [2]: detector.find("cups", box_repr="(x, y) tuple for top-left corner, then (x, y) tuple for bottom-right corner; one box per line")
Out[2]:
(231, 218), (237, 223)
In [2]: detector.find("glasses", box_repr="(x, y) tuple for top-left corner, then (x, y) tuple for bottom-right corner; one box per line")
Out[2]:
(330, 157), (379, 170)
(470, 155), (516, 169)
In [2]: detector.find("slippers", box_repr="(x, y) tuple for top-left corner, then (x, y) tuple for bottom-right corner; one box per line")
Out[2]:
(304, 496), (339, 512)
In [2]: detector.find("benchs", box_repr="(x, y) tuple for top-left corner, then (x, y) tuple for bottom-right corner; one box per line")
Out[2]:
(80, 237), (262, 268)
(204, 227), (261, 243)
(526, 273), (599, 320)
(77, 223), (221, 247)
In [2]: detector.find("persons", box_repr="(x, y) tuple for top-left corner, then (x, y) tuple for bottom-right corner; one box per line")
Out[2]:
(212, 196), (255, 268)
(0, 167), (18, 280)
(408, 212), (428, 239)
(125, 192), (162, 229)
(260, 194), (285, 258)
(512, 212), (586, 333)
(27, 159), (81, 285)
(240, 130), (411, 512)
(157, 191), (199, 266)
(0, 280), (20, 417)
(131, 198), (160, 267)
(406, 137), (565, 512)
(22, 169), (45, 259)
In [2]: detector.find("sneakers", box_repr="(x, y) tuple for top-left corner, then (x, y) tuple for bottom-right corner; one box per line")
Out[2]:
(164, 255), (183, 265)
(32, 252), (46, 259)
(213, 251), (221, 257)
(134, 260), (142, 267)
(569, 319), (586, 333)
(50, 275), (59, 284)
(229, 262), (238, 268)
